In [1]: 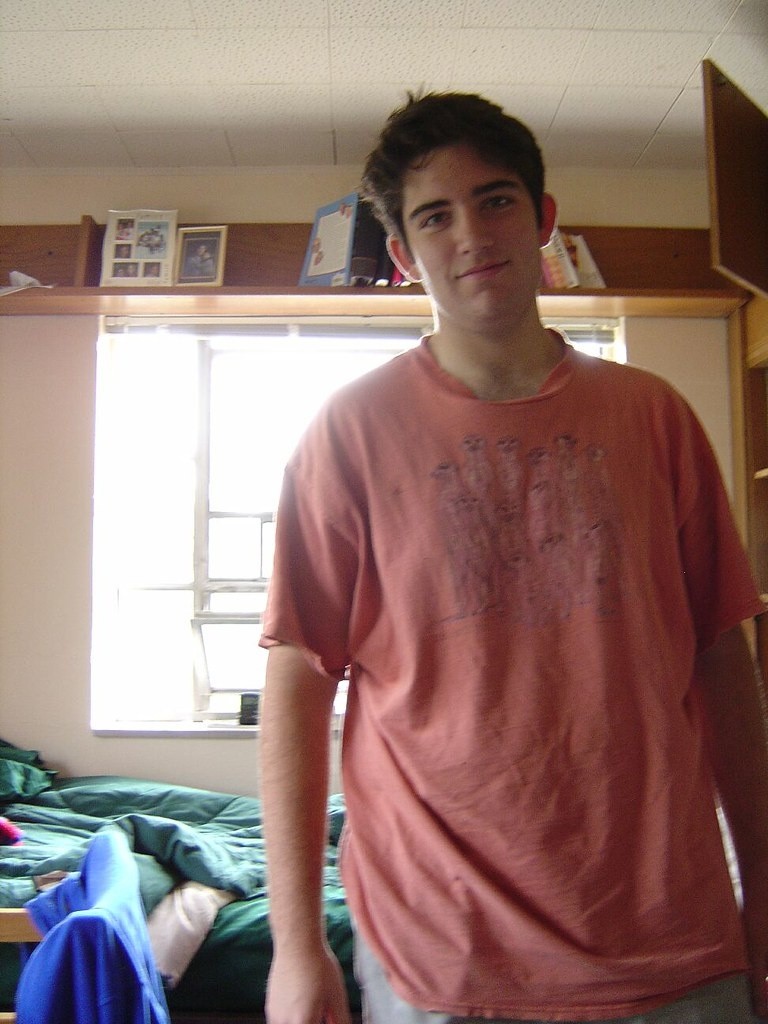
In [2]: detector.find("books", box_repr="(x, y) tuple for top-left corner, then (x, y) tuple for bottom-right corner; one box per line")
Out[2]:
(533, 226), (610, 292)
(295, 191), (414, 288)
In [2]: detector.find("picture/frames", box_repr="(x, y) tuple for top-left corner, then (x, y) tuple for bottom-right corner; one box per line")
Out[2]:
(173, 224), (228, 288)
(99, 208), (179, 288)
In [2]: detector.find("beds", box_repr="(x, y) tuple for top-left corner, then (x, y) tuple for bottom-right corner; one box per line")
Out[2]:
(0, 738), (363, 1024)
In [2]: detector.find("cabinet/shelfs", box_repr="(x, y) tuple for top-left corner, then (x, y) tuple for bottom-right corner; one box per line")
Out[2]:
(701, 58), (768, 708)
(0, 216), (755, 320)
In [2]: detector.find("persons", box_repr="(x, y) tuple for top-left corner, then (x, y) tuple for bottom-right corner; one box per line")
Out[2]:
(251, 86), (766, 1024)
